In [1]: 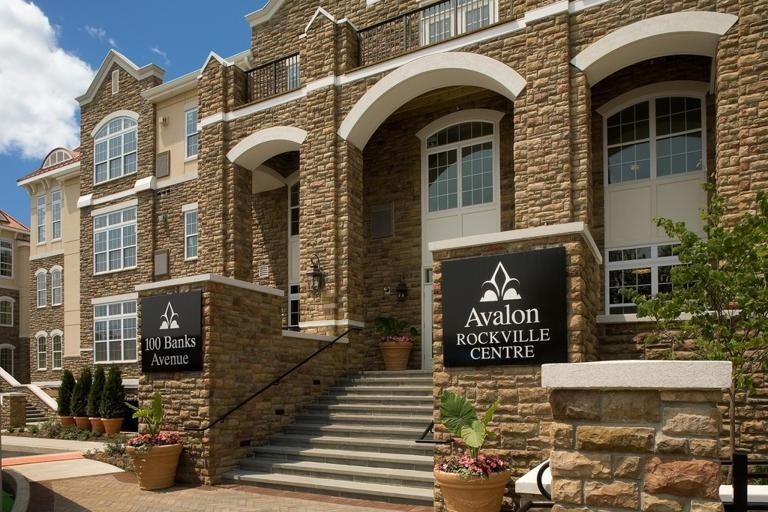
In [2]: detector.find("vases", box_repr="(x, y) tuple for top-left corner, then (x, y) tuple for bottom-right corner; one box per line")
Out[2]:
(126, 444), (183, 491)
(378, 342), (414, 371)
(432, 466), (511, 512)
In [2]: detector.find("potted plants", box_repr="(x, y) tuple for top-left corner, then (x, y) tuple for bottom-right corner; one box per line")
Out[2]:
(56, 362), (125, 436)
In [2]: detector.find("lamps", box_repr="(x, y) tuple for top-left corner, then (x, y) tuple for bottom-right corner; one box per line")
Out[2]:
(396, 280), (410, 301)
(304, 253), (325, 294)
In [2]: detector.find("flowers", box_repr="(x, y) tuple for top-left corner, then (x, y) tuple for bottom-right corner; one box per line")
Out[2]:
(122, 391), (181, 451)
(375, 316), (417, 342)
(431, 389), (515, 481)
